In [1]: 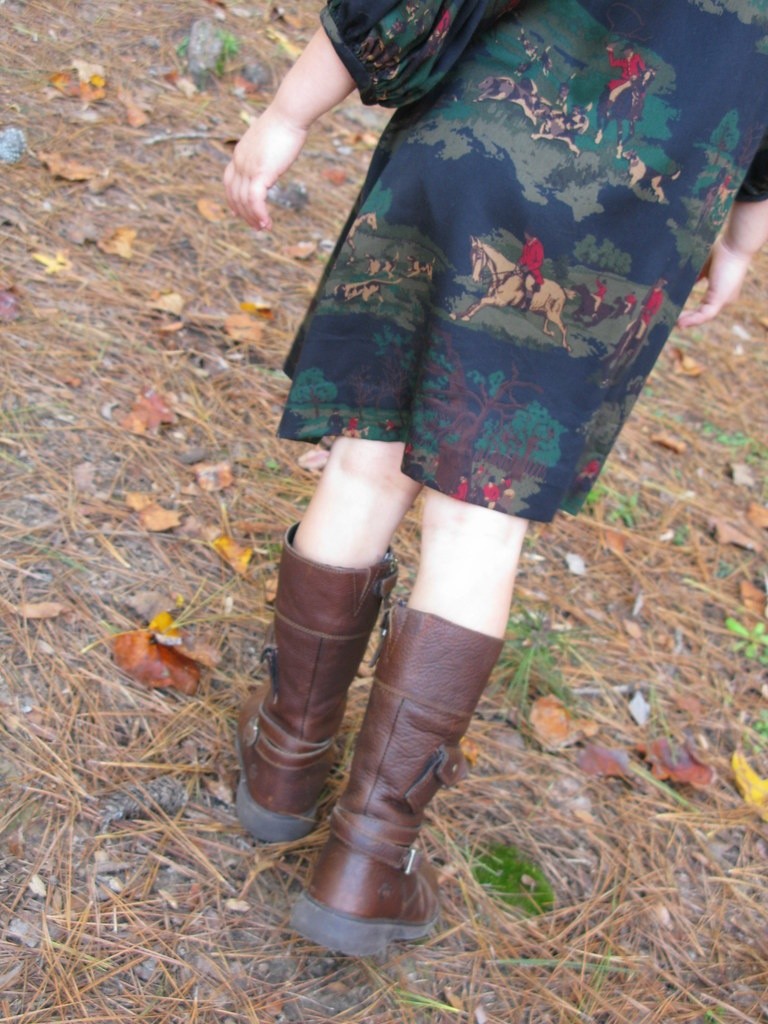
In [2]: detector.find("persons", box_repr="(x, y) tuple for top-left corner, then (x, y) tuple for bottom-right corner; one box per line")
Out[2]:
(222, 0), (768, 957)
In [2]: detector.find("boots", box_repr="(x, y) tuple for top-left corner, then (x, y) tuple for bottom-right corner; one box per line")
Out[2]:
(235, 521), (399, 842)
(290, 598), (505, 955)
